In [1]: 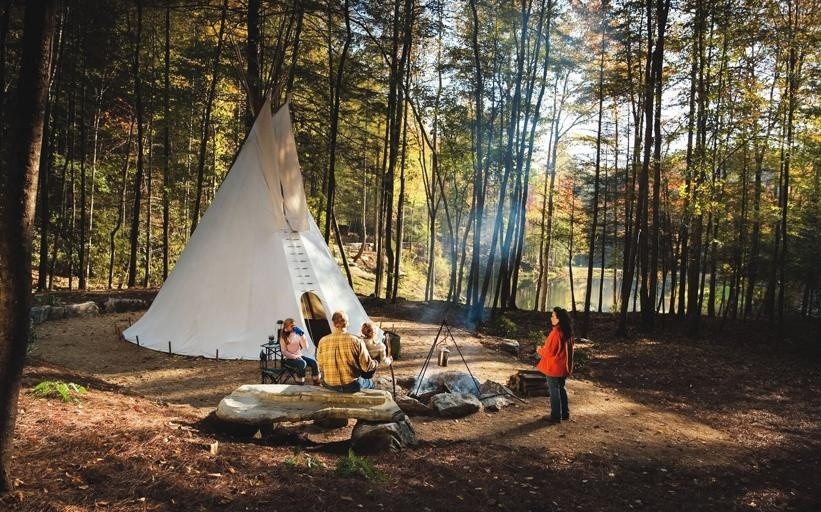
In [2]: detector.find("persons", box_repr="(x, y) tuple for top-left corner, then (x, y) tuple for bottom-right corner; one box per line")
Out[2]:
(536, 307), (574, 424)
(316, 310), (379, 393)
(357, 322), (394, 389)
(280, 318), (321, 387)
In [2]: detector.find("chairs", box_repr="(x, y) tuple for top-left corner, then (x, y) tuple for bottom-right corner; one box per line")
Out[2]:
(281, 344), (304, 387)
(258, 352), (283, 384)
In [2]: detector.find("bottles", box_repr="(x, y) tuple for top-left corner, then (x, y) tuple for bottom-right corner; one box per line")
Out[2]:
(439, 347), (451, 366)
(293, 326), (303, 337)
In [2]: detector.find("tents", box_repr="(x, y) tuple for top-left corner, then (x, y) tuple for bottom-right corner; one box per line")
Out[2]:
(122, 91), (385, 360)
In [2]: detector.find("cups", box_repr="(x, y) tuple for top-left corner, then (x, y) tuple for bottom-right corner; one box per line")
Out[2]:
(268, 336), (274, 344)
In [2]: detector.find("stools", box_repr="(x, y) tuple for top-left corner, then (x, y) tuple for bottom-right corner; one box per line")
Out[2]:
(262, 342), (284, 367)
